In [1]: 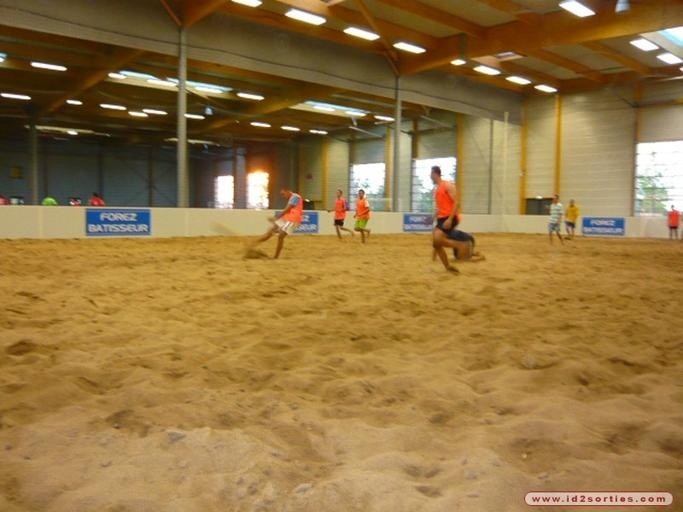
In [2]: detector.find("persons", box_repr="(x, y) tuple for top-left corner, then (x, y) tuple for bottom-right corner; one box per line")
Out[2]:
(429, 166), (471, 272)
(66, 195), (82, 205)
(563, 199), (579, 241)
(545, 195), (565, 246)
(40, 195), (58, 206)
(325, 187), (354, 240)
(667, 205), (681, 239)
(87, 192), (105, 207)
(352, 189), (373, 243)
(430, 227), (486, 263)
(254, 186), (304, 261)
